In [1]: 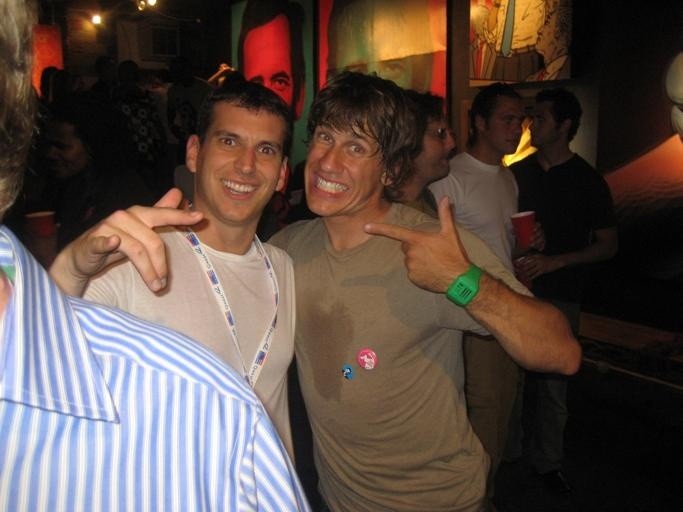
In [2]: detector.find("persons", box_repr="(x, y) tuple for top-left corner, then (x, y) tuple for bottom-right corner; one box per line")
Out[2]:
(265, 69), (583, 512)
(326, 55), (434, 95)
(1, 1), (312, 512)
(236, 0), (306, 123)
(509, 87), (619, 497)
(472, 1), (573, 81)
(427, 81), (526, 498)
(48, 78), (297, 477)
(386, 88), (455, 220)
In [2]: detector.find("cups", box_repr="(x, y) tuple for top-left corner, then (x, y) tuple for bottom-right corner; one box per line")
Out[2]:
(512, 256), (533, 289)
(26, 210), (59, 237)
(511, 211), (537, 251)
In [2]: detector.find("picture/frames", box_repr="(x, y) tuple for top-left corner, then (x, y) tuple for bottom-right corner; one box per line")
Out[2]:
(314, 0), (453, 128)
(226, 0), (316, 215)
(462, 0), (578, 88)
(460, 96), (538, 154)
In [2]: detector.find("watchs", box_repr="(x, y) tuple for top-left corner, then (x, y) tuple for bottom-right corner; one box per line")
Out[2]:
(446, 264), (481, 307)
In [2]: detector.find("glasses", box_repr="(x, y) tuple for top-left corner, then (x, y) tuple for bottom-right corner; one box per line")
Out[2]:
(423, 126), (451, 139)
